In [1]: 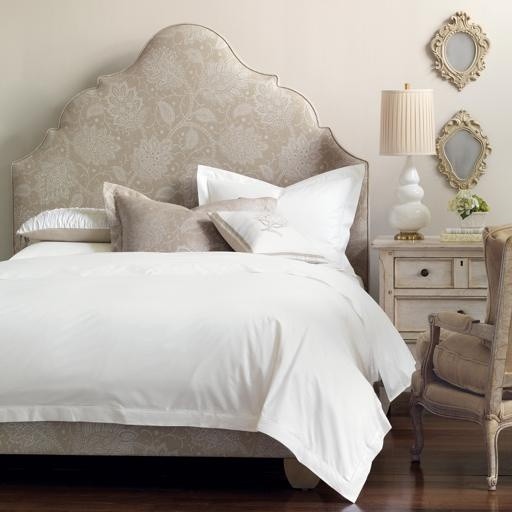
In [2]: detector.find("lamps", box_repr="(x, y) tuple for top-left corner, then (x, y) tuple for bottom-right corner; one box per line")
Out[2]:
(379, 82), (436, 241)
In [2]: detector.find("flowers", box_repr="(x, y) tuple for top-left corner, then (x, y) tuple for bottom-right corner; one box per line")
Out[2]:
(449, 191), (492, 220)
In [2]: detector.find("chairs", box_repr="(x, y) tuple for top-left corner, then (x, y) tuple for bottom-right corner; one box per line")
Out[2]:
(410, 223), (512, 491)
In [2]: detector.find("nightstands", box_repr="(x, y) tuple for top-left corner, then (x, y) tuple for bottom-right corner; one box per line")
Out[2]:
(368, 234), (491, 420)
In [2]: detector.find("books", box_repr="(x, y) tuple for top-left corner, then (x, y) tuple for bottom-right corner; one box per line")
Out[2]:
(445, 227), (484, 234)
(441, 233), (483, 243)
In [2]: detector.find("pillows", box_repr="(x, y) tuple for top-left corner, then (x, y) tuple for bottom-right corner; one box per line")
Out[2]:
(206, 208), (328, 258)
(15, 206), (115, 241)
(194, 161), (366, 260)
(101, 180), (277, 252)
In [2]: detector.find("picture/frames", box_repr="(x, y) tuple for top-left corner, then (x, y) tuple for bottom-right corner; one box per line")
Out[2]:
(434, 109), (495, 192)
(429, 10), (492, 94)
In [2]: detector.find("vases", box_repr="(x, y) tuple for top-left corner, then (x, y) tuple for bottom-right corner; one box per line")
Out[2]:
(461, 212), (489, 227)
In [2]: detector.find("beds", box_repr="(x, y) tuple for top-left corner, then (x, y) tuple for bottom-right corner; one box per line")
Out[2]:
(0, 22), (418, 505)
